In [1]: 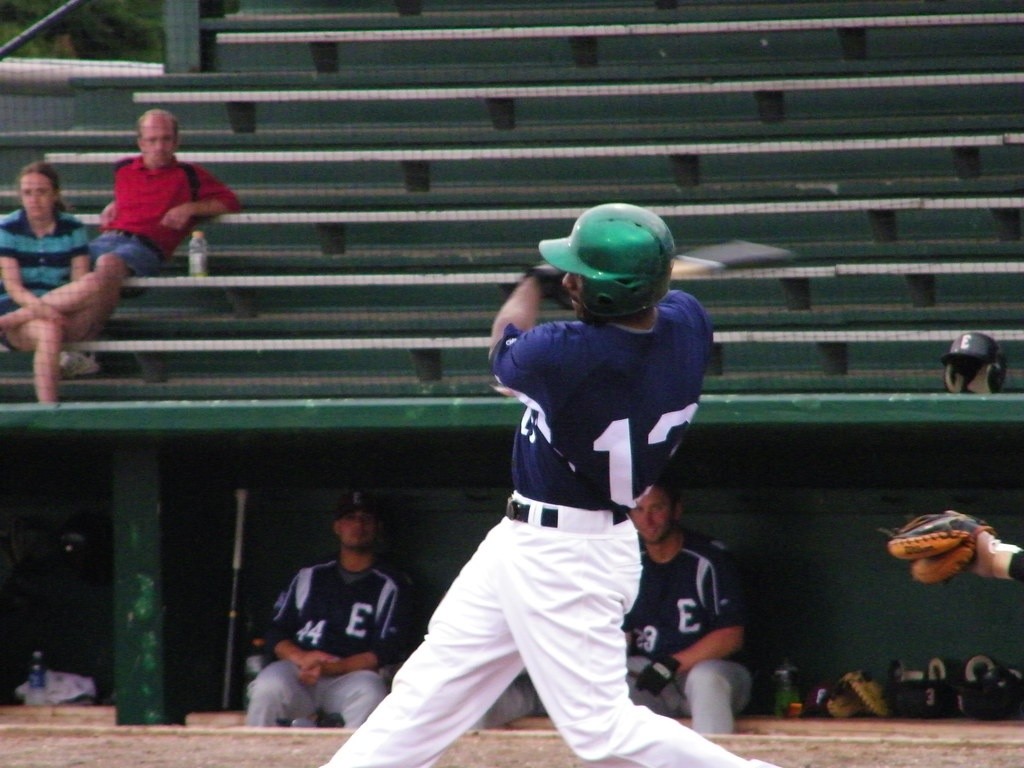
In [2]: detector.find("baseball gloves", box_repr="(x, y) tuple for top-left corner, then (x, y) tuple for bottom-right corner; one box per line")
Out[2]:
(827, 670), (889, 721)
(886, 509), (989, 590)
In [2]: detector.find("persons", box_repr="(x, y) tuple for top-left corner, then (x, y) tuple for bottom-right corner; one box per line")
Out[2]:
(244, 490), (399, 728)
(321, 204), (777, 768)
(622, 479), (754, 735)
(945, 509), (1023, 581)
(0, 162), (123, 403)
(87, 110), (241, 279)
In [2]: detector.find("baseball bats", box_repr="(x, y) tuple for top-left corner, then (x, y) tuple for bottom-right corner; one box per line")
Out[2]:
(669, 238), (788, 283)
(222, 486), (249, 710)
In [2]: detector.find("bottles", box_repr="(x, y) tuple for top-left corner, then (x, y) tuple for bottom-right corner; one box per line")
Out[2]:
(772, 662), (796, 719)
(245, 638), (262, 683)
(187, 231), (209, 277)
(27, 651), (46, 705)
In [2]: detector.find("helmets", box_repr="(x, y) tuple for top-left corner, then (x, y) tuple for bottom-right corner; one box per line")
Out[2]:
(538, 202), (676, 317)
(940, 332), (1008, 395)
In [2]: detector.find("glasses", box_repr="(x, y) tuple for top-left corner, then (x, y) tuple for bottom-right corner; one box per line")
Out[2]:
(344, 513), (374, 522)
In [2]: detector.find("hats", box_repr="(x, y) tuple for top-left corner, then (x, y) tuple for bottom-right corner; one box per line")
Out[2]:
(335, 490), (377, 518)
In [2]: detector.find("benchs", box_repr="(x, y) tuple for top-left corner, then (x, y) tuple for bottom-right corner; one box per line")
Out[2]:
(0, 0), (1024, 723)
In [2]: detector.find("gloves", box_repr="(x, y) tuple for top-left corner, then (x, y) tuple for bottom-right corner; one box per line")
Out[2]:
(634, 655), (681, 697)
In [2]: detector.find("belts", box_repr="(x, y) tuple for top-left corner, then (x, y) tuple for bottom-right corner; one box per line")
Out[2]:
(114, 230), (166, 262)
(505, 497), (628, 529)
